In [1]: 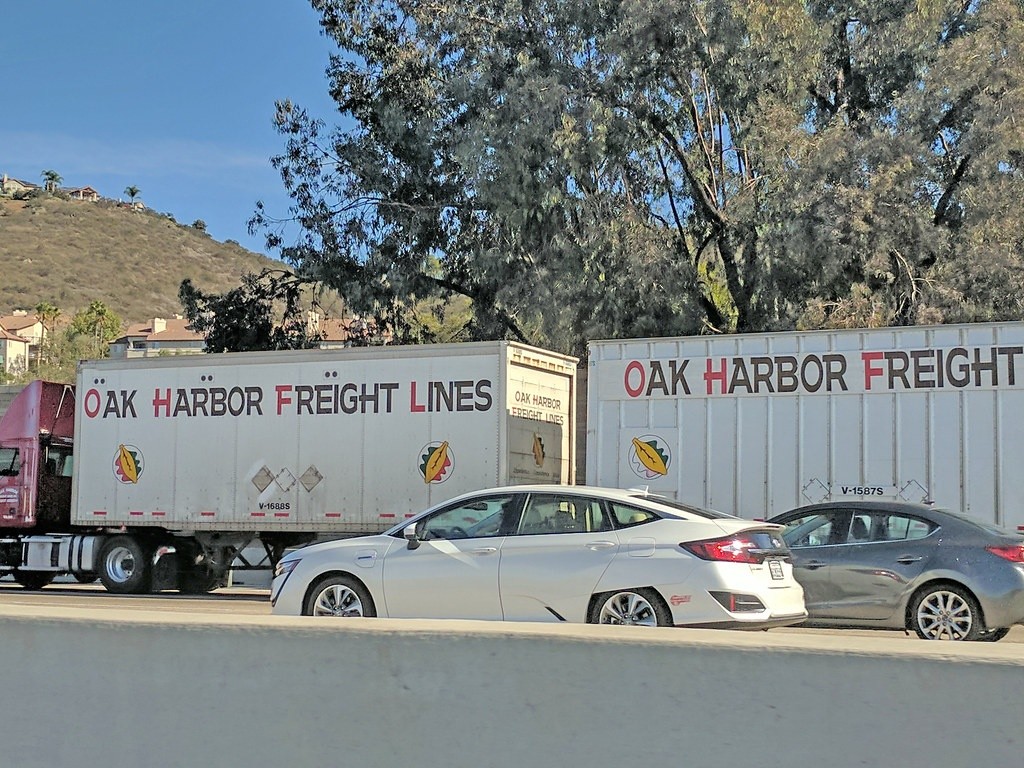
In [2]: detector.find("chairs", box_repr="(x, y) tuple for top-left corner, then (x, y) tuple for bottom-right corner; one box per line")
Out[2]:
(849, 518), (869, 542)
(526, 508), (542, 526)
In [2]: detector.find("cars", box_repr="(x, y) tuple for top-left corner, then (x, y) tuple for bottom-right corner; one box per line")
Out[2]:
(757, 500), (1024, 643)
(268, 483), (811, 630)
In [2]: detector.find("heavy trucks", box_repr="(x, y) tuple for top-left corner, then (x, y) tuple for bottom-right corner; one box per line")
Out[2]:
(1, 339), (577, 598)
(577, 320), (1024, 544)
